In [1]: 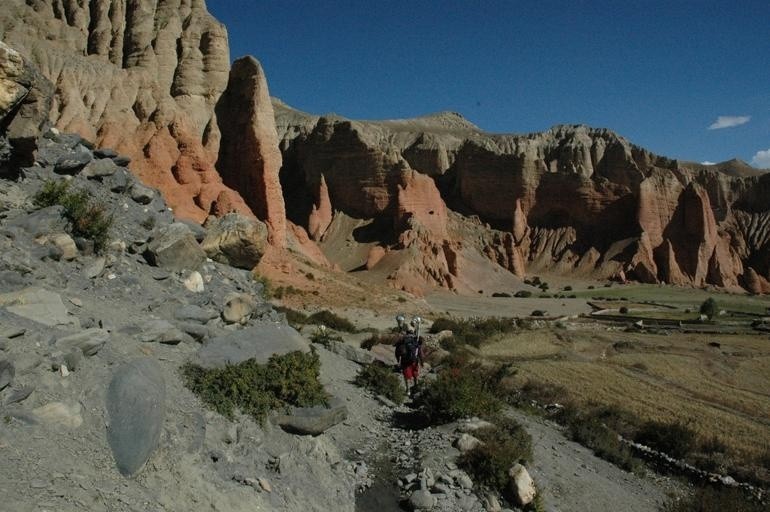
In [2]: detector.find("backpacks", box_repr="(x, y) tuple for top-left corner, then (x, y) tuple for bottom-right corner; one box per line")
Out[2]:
(404, 337), (418, 361)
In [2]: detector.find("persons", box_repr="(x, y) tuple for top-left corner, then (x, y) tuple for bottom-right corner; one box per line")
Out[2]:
(410, 320), (424, 368)
(397, 327), (425, 397)
